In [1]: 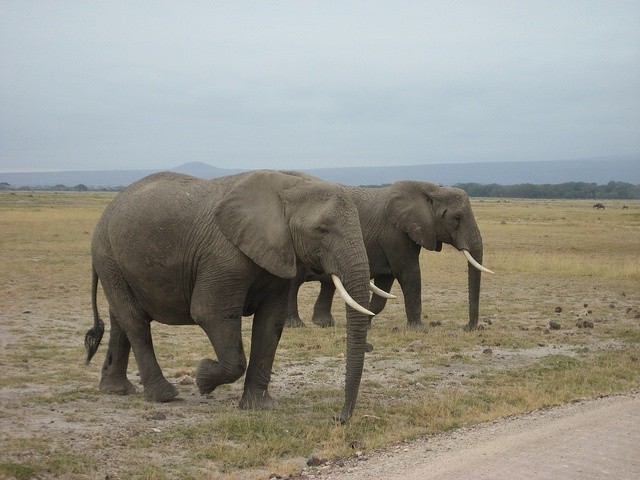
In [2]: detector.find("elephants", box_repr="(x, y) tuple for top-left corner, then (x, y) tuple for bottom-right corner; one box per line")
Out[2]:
(84, 168), (399, 426)
(284, 180), (495, 332)
